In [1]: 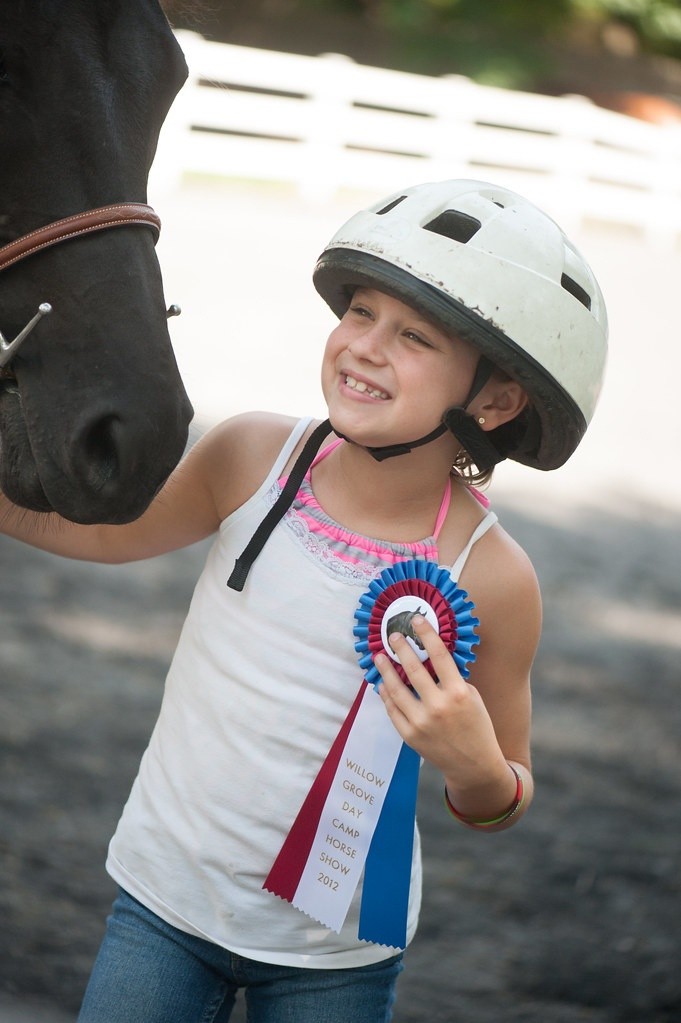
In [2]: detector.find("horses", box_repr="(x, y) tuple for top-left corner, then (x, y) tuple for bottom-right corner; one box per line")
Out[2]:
(2, 0), (200, 531)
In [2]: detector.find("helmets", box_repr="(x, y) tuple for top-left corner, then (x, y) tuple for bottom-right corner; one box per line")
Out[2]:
(312, 179), (609, 471)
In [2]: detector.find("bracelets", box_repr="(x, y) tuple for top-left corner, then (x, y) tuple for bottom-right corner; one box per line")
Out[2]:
(445, 765), (526, 828)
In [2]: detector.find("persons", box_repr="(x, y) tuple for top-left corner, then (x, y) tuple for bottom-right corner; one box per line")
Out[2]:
(0, 177), (609, 1023)
